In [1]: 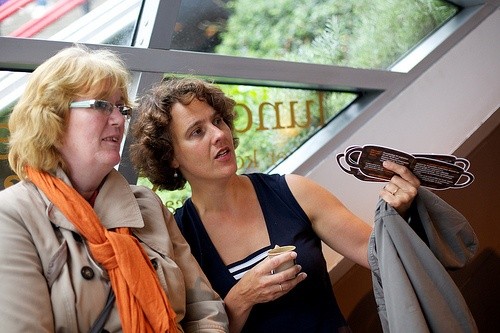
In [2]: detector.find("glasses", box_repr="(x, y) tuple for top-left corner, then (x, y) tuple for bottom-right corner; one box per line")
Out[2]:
(68, 99), (133, 119)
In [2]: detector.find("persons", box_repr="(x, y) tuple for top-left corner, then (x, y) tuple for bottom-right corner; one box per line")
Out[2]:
(0, 45), (227, 333)
(131, 79), (420, 333)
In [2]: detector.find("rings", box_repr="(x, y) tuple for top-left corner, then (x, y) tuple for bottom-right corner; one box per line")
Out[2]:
(279, 284), (285, 293)
(391, 187), (399, 195)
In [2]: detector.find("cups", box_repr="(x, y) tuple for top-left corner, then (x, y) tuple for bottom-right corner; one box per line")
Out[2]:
(267, 246), (298, 283)
